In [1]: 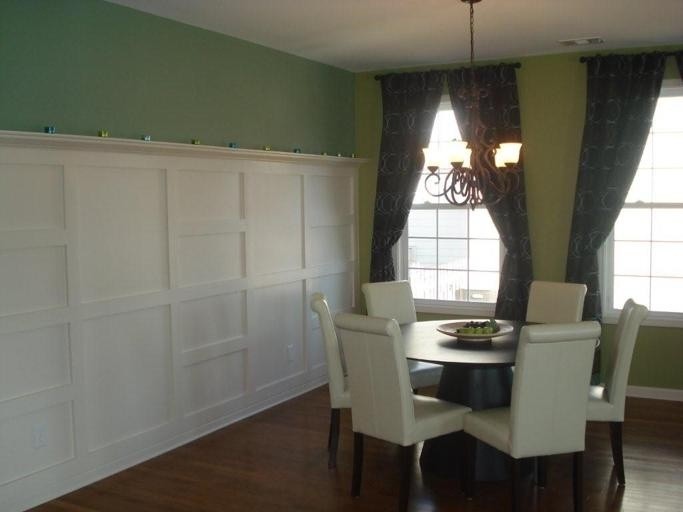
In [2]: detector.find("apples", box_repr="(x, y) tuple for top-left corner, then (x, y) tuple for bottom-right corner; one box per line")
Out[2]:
(469, 326), (492, 334)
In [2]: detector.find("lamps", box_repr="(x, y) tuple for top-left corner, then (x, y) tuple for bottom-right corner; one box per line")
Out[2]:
(422, 1), (523, 210)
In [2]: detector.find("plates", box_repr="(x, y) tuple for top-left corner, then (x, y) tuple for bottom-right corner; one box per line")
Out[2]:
(436, 321), (514, 342)
